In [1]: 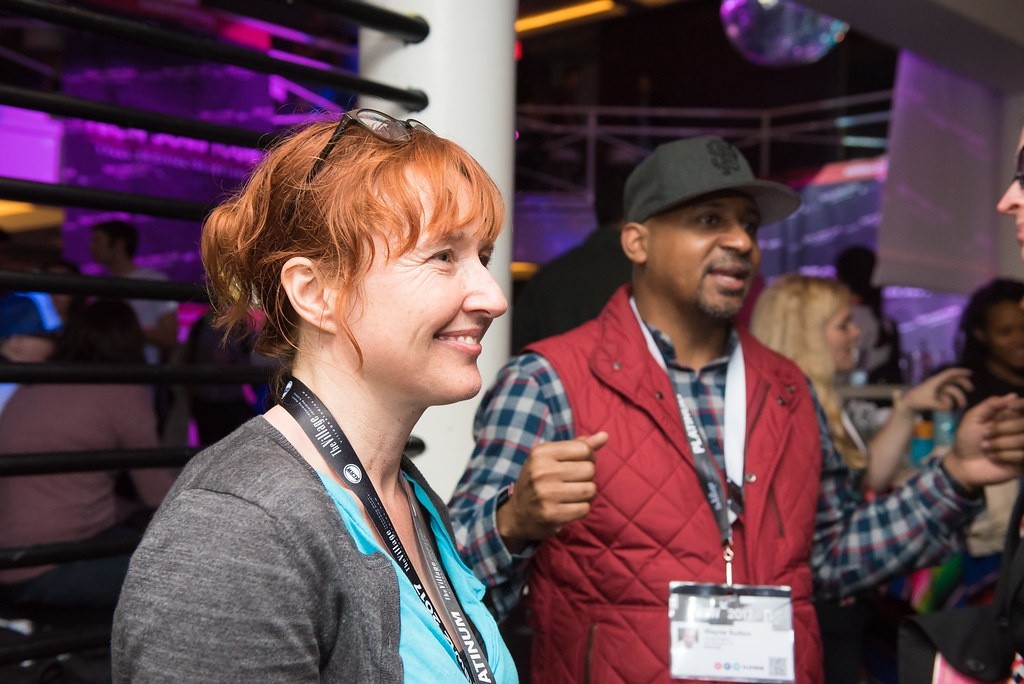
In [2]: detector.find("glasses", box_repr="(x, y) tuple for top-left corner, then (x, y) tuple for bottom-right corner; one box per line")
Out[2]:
(1013, 145), (1024, 189)
(288, 107), (432, 223)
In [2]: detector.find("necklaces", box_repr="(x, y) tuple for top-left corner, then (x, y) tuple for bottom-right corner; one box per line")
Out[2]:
(276, 373), (502, 684)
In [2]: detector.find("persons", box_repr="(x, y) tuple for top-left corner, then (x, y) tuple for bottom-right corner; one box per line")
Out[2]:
(106, 108), (525, 683)
(2, 211), (281, 681)
(751, 246), (1024, 683)
(993, 127), (1023, 250)
(445, 141), (1023, 684)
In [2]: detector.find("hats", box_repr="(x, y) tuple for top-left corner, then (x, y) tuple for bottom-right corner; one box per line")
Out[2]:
(623, 136), (802, 224)
